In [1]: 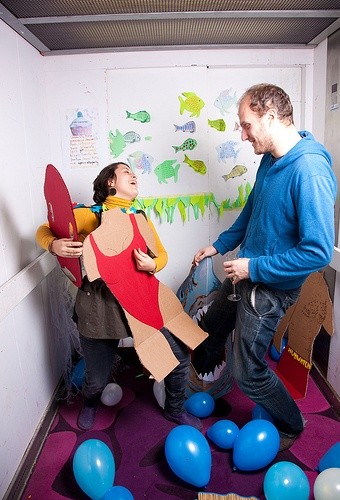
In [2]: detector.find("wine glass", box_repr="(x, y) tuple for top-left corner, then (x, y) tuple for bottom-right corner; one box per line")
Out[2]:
(225, 250), (242, 302)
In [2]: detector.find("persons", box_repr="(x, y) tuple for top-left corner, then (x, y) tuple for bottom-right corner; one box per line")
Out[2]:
(35, 162), (204, 431)
(191, 84), (339, 451)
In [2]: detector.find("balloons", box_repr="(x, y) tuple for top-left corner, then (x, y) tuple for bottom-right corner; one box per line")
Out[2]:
(69, 338), (340, 500)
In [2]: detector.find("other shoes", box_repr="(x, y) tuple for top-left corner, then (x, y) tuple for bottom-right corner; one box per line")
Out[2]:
(163, 411), (204, 430)
(278, 431), (297, 449)
(77, 406), (96, 430)
